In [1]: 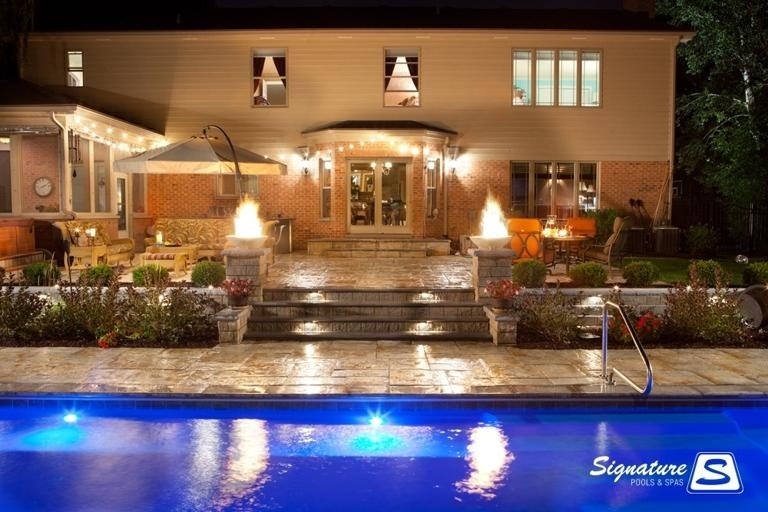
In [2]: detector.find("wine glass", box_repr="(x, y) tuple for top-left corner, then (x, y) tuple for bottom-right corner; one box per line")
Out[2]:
(555, 219), (569, 231)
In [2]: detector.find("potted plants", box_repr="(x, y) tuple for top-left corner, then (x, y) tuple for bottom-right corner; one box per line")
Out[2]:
(219, 278), (254, 310)
(486, 281), (522, 313)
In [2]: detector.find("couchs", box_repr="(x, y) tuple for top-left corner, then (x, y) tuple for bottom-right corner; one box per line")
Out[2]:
(145, 217), (235, 266)
(52, 220), (134, 273)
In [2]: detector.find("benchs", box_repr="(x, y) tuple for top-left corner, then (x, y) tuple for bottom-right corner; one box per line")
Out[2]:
(141, 252), (187, 278)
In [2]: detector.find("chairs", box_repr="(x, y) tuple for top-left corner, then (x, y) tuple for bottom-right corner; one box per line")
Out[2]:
(578, 215), (634, 280)
(555, 215), (599, 263)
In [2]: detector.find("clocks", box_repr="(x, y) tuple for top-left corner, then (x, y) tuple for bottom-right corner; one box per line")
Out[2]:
(34, 177), (53, 196)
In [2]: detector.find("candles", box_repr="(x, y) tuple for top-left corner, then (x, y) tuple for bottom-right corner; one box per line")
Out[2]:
(156, 232), (162, 243)
(90, 229), (95, 237)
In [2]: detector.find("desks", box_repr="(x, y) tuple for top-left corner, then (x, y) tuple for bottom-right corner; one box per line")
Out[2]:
(64, 245), (107, 278)
(541, 236), (592, 277)
(145, 243), (197, 273)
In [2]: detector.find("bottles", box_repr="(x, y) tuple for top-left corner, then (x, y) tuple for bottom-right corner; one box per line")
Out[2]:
(545, 215), (558, 232)
